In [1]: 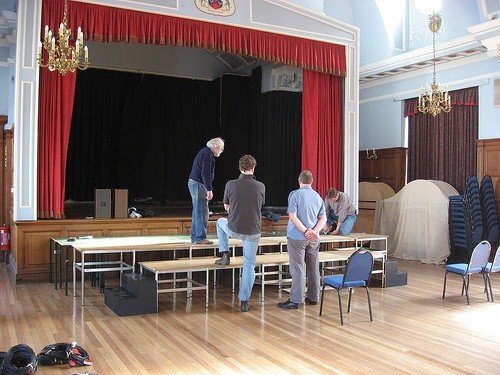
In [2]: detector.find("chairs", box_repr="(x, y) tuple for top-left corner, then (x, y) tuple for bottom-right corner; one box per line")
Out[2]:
(318, 252), (373, 326)
(443, 175), (500, 305)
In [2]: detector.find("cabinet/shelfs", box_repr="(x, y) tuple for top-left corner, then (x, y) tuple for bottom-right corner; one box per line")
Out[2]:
(359, 147), (408, 194)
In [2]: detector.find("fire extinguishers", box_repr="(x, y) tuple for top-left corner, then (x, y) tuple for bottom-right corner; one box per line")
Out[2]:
(0, 222), (10, 251)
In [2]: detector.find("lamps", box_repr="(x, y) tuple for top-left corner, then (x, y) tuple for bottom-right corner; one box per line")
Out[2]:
(417, 11), (452, 116)
(36, 0), (91, 77)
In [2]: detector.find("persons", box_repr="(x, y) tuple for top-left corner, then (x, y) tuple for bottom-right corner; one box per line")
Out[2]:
(215, 155), (265, 312)
(188, 138), (224, 244)
(278, 170), (327, 309)
(323, 188), (356, 235)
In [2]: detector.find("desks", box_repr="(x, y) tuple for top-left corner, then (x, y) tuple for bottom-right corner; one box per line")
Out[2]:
(49, 233), (388, 312)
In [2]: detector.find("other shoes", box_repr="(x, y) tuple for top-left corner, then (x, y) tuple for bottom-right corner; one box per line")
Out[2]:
(215, 252), (231, 265)
(305, 297), (316, 305)
(199, 240), (214, 244)
(278, 299), (298, 309)
(241, 301), (250, 311)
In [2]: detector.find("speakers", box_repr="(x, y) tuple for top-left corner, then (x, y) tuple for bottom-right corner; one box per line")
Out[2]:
(94, 188), (128, 218)
